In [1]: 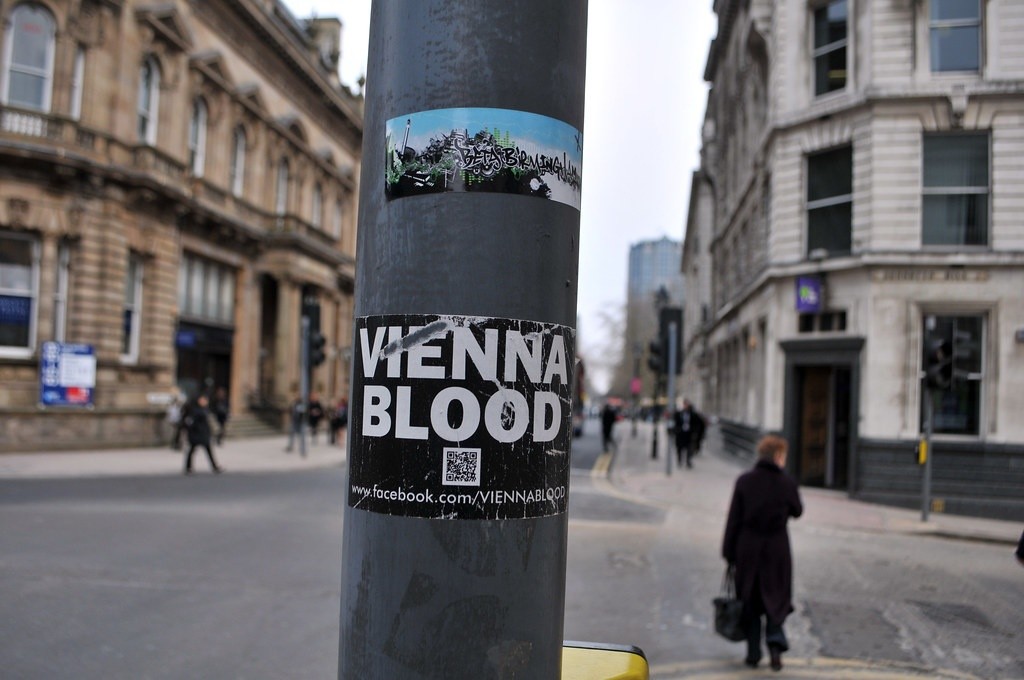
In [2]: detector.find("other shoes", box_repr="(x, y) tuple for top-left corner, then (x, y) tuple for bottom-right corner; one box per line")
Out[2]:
(770, 659), (782, 670)
(744, 656), (759, 668)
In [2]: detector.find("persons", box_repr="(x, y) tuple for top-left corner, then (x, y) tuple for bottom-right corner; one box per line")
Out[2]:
(598, 403), (618, 453)
(667, 398), (709, 466)
(722, 436), (804, 673)
(169, 378), (348, 475)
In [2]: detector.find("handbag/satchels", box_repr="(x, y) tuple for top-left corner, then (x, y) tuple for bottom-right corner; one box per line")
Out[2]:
(712, 564), (747, 641)
